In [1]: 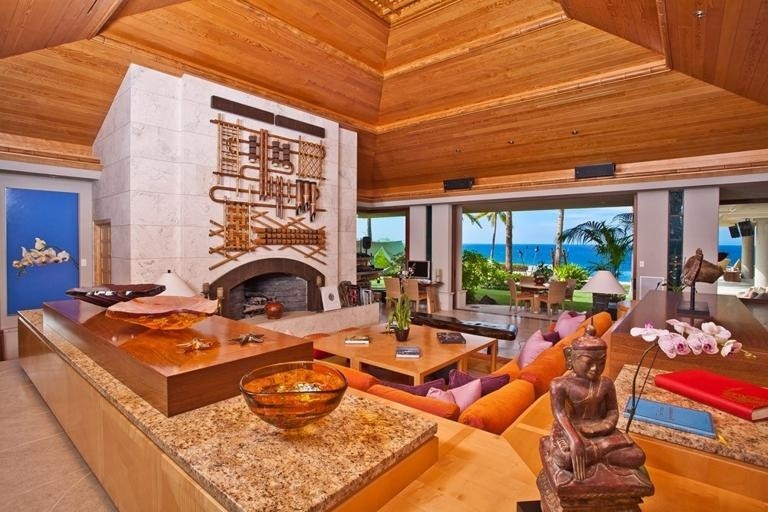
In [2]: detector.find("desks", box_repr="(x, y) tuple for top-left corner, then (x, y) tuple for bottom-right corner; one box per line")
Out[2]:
(419, 282), (443, 314)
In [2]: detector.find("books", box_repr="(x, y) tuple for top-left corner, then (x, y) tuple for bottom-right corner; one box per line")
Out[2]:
(437, 332), (466, 344)
(654, 367), (768, 422)
(624, 396), (715, 438)
(396, 346), (420, 358)
(345, 335), (369, 344)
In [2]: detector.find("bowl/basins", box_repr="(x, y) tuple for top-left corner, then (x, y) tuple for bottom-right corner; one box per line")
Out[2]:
(106, 295), (219, 331)
(116, 326), (221, 367)
(80, 308), (145, 340)
(723, 272), (740, 281)
(236, 359), (349, 431)
(63, 283), (165, 306)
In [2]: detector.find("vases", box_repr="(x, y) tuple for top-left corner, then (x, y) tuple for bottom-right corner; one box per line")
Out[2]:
(265, 302), (283, 319)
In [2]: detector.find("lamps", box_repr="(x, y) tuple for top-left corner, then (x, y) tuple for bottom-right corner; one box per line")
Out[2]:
(581, 271), (628, 314)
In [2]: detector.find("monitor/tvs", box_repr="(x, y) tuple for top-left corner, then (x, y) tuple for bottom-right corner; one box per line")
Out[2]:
(407, 260), (430, 279)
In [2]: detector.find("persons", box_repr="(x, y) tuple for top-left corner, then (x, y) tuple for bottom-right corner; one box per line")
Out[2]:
(549, 316), (646, 470)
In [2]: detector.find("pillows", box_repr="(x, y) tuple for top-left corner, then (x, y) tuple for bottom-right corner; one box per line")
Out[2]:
(379, 311), (591, 411)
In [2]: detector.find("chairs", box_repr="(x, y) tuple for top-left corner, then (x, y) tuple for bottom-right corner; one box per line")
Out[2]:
(383, 277), (427, 311)
(508, 275), (576, 316)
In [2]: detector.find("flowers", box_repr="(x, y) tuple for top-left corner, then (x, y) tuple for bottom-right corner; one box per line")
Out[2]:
(12, 236), (79, 276)
(622, 317), (742, 433)
(386, 257), (416, 330)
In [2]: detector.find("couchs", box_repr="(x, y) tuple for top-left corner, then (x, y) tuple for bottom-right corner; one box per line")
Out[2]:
(301, 311), (612, 435)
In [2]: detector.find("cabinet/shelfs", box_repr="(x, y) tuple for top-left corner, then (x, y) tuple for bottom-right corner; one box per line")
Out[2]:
(18, 290), (768, 512)
